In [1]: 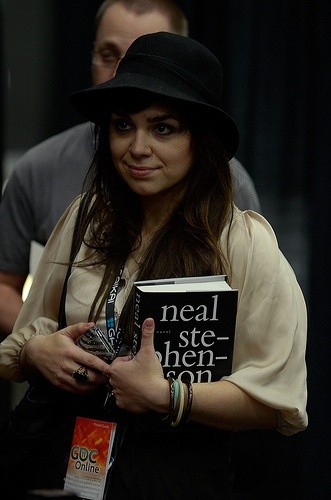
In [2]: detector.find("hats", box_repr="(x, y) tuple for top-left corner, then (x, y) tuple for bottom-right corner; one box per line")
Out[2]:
(67, 31), (239, 165)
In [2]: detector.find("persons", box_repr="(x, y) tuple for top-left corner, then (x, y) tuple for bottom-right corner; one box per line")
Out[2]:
(0, 30), (311, 499)
(1, 0), (262, 337)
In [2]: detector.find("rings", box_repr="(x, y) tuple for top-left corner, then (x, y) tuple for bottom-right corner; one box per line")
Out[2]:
(112, 389), (116, 398)
(72, 366), (89, 383)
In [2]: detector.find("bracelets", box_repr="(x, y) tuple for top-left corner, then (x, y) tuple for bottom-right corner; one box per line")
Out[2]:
(160, 377), (193, 427)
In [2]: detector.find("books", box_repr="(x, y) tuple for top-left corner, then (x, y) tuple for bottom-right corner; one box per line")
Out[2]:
(131, 275), (239, 383)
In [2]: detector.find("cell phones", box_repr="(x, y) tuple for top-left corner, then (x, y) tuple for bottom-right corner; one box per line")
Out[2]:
(75, 327), (116, 364)
